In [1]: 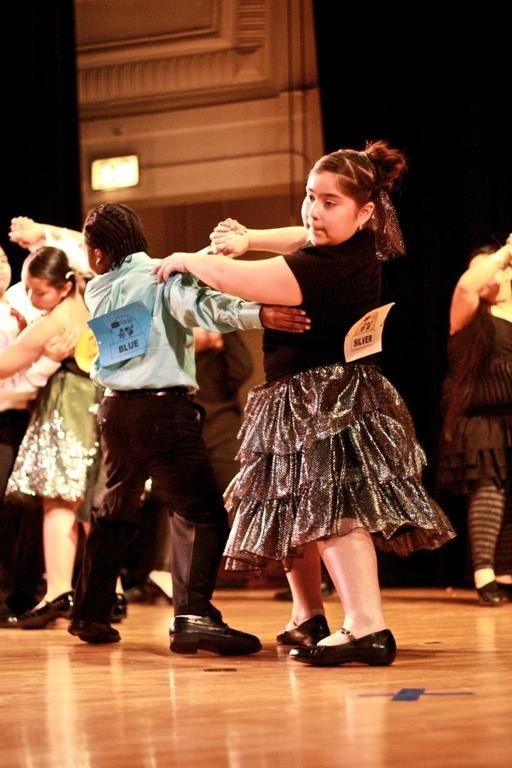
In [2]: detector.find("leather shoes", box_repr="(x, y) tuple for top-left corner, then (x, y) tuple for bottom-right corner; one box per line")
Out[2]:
(170, 616), (261, 654)
(479, 590), (512, 606)
(9, 590), (126, 643)
(277, 615), (396, 665)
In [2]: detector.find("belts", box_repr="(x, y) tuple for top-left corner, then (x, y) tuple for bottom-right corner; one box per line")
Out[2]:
(102, 388), (186, 396)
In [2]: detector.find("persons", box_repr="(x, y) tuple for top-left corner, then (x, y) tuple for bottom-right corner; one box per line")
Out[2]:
(432, 230), (512, 605)
(1, 216), (87, 630)
(66, 200), (314, 656)
(0, 213), (128, 629)
(151, 138), (458, 668)
(119, 330), (255, 605)
